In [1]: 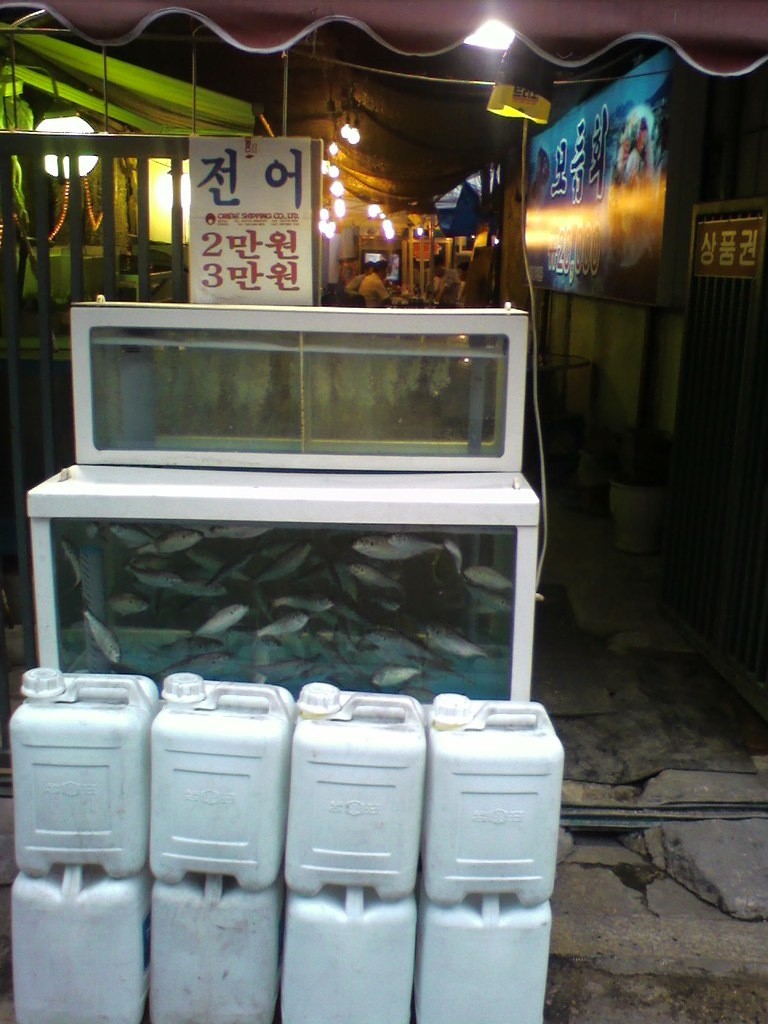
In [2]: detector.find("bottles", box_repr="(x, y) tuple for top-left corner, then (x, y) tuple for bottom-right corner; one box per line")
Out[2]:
(8, 667), (566, 1024)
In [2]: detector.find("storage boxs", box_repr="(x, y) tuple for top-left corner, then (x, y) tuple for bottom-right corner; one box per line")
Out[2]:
(26, 299), (545, 701)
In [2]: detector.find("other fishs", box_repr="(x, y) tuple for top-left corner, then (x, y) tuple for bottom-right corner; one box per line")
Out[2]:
(58, 521), (515, 705)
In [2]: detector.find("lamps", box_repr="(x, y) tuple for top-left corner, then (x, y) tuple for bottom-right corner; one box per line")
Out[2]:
(15, 63), (105, 180)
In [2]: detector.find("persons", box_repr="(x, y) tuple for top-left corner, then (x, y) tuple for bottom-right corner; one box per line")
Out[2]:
(457, 262), (470, 302)
(344, 261), (375, 294)
(426, 255), (456, 297)
(358, 260), (392, 306)
